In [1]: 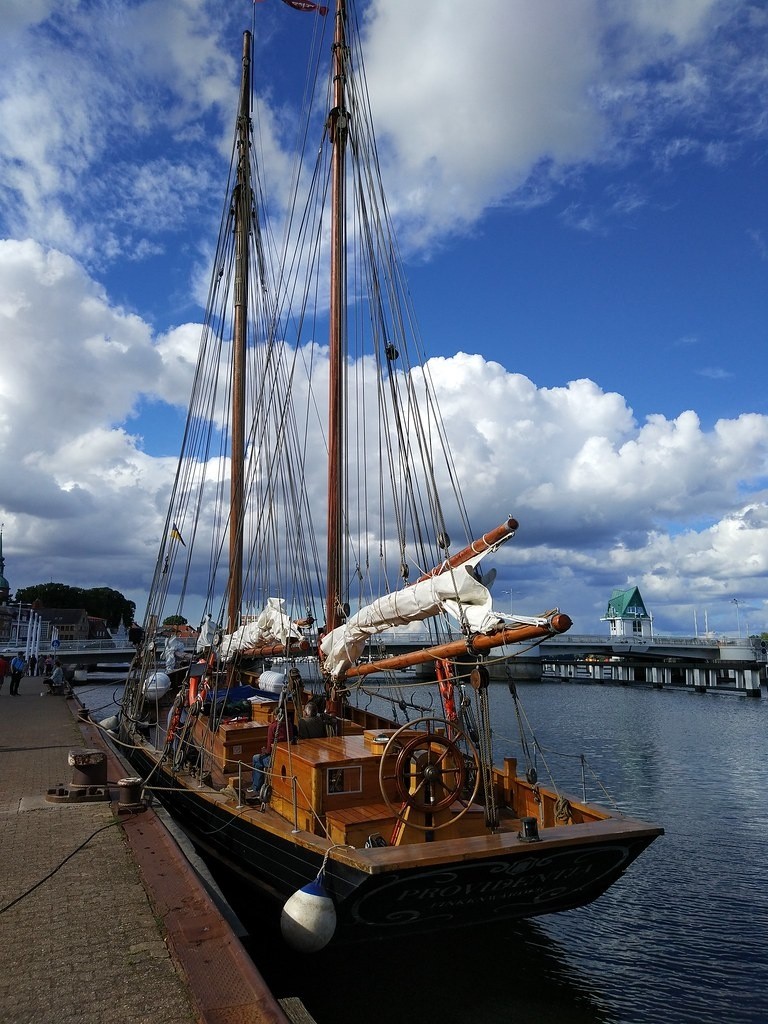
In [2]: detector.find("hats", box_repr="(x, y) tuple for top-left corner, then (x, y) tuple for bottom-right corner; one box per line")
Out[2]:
(18, 651), (26, 655)
(53, 662), (61, 667)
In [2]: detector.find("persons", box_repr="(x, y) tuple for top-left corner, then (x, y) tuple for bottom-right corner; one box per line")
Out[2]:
(246, 707), (293, 792)
(29, 654), (63, 694)
(299, 702), (328, 739)
(0, 651), (26, 697)
(167, 689), (195, 751)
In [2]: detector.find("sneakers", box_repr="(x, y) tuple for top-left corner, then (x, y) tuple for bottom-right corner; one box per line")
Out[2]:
(247, 787), (254, 793)
(10, 693), (22, 698)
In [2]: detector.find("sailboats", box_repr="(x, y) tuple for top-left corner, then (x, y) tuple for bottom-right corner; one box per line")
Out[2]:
(97, 0), (670, 942)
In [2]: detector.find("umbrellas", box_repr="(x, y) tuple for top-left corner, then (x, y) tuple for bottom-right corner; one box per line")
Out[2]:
(164, 627), (181, 632)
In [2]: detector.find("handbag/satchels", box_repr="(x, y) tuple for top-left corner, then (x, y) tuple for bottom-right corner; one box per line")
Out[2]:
(43, 680), (54, 685)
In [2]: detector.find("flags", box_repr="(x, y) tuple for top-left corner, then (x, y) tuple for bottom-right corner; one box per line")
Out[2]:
(171, 523), (186, 547)
